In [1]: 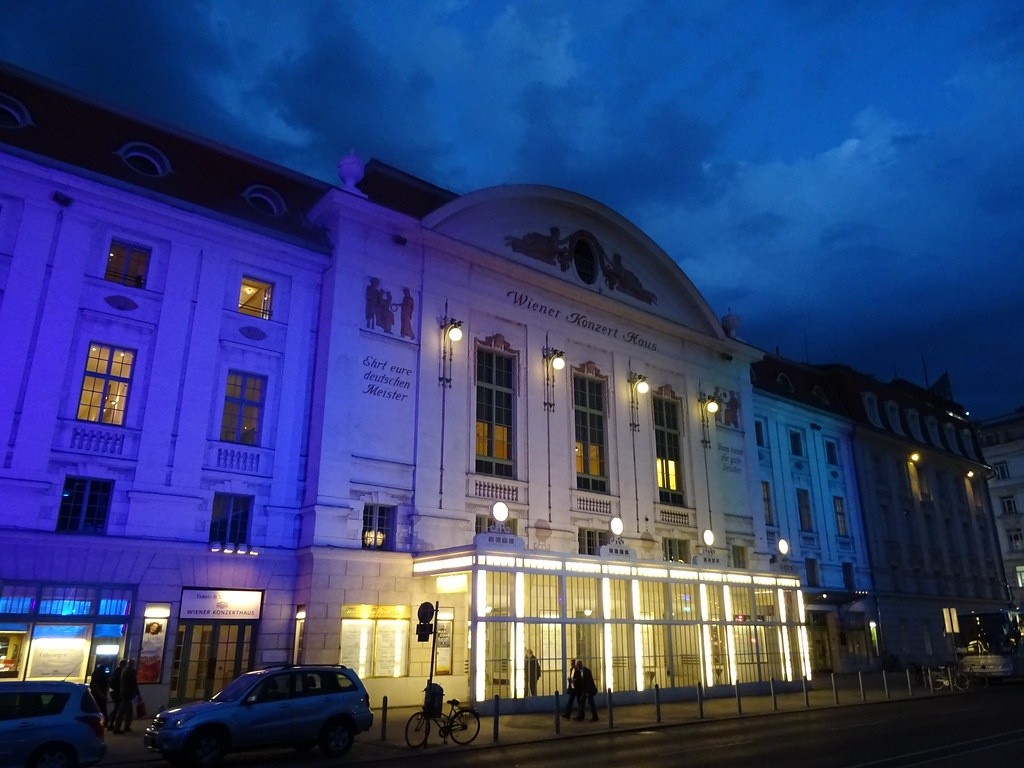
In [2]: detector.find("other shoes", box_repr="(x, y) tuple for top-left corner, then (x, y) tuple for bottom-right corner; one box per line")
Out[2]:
(574, 716), (585, 721)
(124, 727), (131, 731)
(108, 725), (114, 730)
(589, 717), (598, 721)
(562, 714), (570, 719)
(113, 728), (124, 734)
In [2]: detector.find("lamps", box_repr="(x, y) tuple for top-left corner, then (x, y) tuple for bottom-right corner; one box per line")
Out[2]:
(236, 543), (247, 554)
(210, 541), (222, 552)
(697, 391), (720, 449)
(249, 547), (261, 556)
(630, 373), (650, 431)
(440, 316), (463, 387)
(224, 543), (234, 554)
(544, 349), (566, 413)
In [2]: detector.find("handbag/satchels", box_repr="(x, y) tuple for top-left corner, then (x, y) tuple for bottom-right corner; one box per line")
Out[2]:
(136, 701), (146, 718)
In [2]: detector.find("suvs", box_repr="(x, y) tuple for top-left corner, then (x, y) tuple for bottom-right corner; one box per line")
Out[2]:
(0, 680), (108, 767)
(145, 660), (375, 767)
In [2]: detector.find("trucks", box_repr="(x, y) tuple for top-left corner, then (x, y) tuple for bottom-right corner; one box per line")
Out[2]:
(961, 640), (1013, 679)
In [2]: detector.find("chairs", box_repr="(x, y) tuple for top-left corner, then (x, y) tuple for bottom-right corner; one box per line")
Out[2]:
(307, 676), (317, 689)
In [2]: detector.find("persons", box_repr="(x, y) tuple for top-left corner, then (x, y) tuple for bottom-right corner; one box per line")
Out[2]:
(90, 657), (141, 735)
(524, 648), (540, 697)
(561, 657), (600, 721)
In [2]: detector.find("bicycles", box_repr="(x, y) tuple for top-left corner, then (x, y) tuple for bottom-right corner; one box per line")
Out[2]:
(404, 683), (480, 748)
(927, 660), (970, 691)
